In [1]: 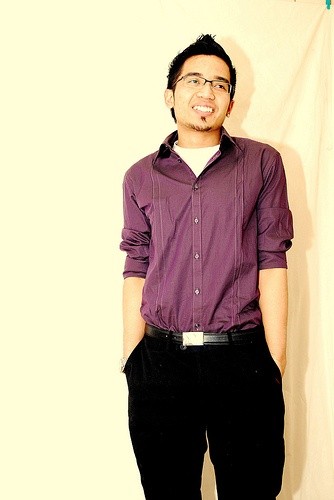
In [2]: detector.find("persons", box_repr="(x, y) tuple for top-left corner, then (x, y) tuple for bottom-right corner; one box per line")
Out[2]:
(118, 28), (294, 500)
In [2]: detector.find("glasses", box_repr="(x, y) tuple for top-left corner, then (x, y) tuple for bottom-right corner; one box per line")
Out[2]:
(169, 75), (233, 97)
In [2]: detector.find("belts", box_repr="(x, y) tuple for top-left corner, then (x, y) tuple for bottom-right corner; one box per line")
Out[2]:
(144, 323), (265, 348)
